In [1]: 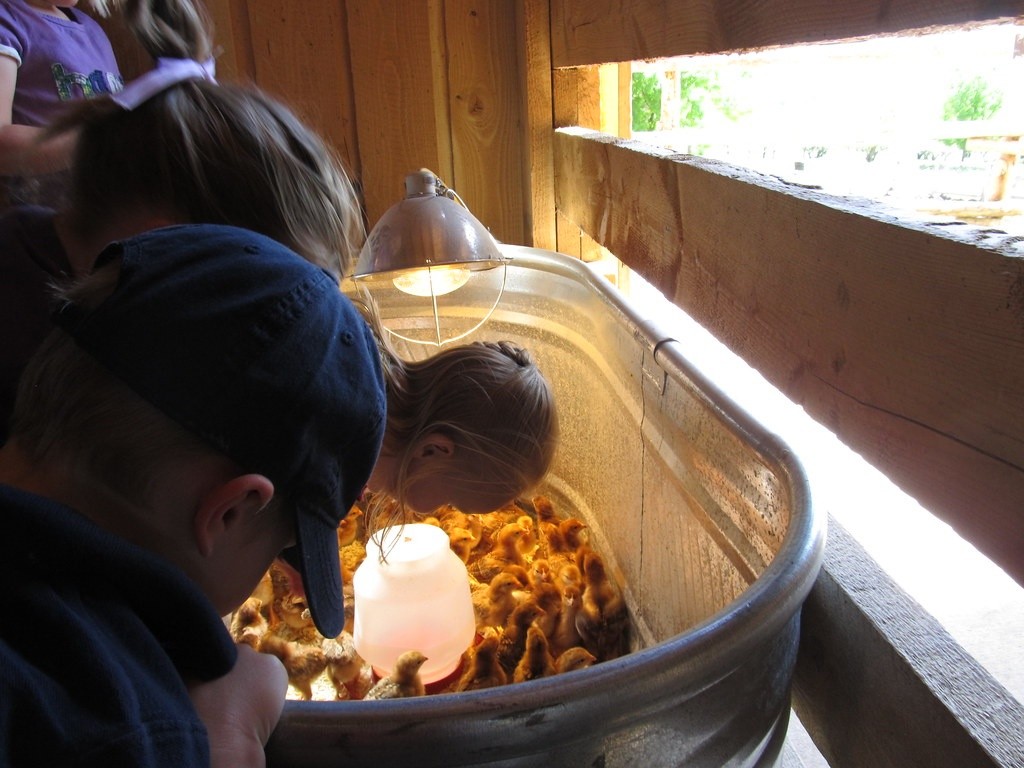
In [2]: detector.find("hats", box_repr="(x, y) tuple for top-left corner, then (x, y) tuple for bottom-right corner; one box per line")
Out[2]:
(62, 225), (387, 640)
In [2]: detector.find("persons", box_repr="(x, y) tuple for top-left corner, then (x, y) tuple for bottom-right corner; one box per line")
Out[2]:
(0, 226), (387, 768)
(0, 1), (356, 440)
(0, 0), (123, 206)
(353, 290), (559, 514)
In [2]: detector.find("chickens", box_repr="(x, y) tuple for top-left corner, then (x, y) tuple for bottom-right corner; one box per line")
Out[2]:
(228, 479), (629, 702)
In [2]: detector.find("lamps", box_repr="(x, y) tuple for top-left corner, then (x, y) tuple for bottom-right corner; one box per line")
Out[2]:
(345, 168), (512, 348)
